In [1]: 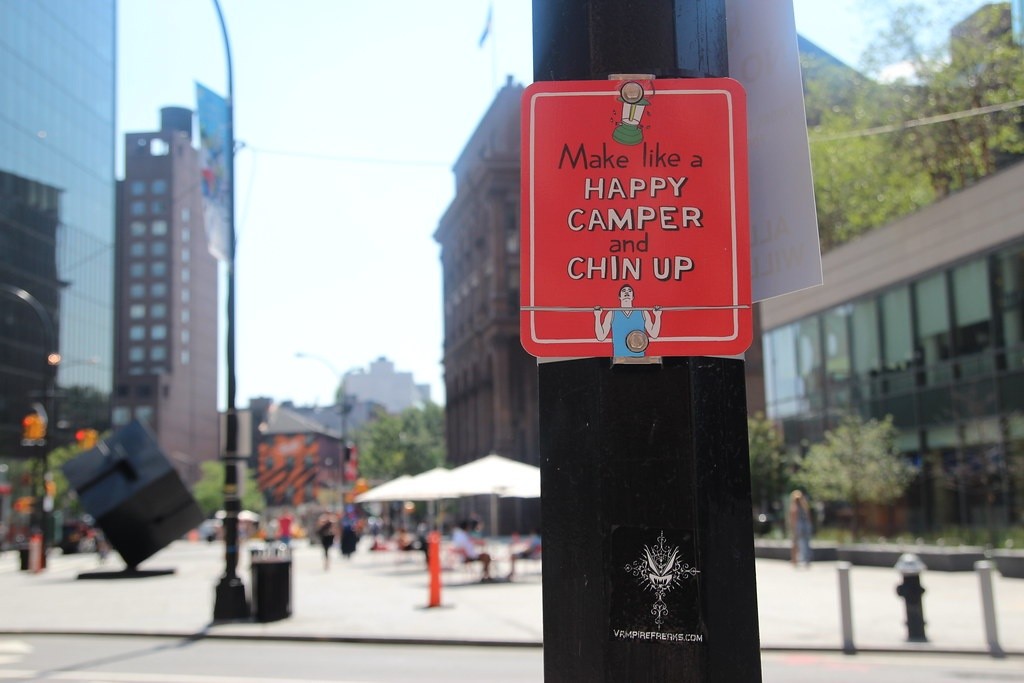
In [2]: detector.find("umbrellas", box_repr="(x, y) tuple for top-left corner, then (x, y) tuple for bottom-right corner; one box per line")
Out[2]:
(391, 467), (451, 536)
(353, 475), (413, 535)
(436, 454), (540, 535)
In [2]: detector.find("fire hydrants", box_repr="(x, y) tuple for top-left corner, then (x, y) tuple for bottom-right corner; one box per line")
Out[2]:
(893, 554), (930, 642)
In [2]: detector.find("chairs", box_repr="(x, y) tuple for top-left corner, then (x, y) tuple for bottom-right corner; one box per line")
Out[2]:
(451, 538), (542, 583)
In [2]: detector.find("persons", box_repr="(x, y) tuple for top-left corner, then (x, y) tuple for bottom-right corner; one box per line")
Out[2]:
(278, 507), (292, 547)
(507, 529), (540, 581)
(398, 530), (415, 551)
(264, 520), (276, 543)
(790, 491), (811, 566)
(315, 518), (334, 561)
(340, 521), (357, 556)
(452, 521), (493, 583)
(469, 519), (481, 539)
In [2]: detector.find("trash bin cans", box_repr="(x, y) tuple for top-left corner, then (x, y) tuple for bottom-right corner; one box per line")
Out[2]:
(247, 544), (296, 622)
